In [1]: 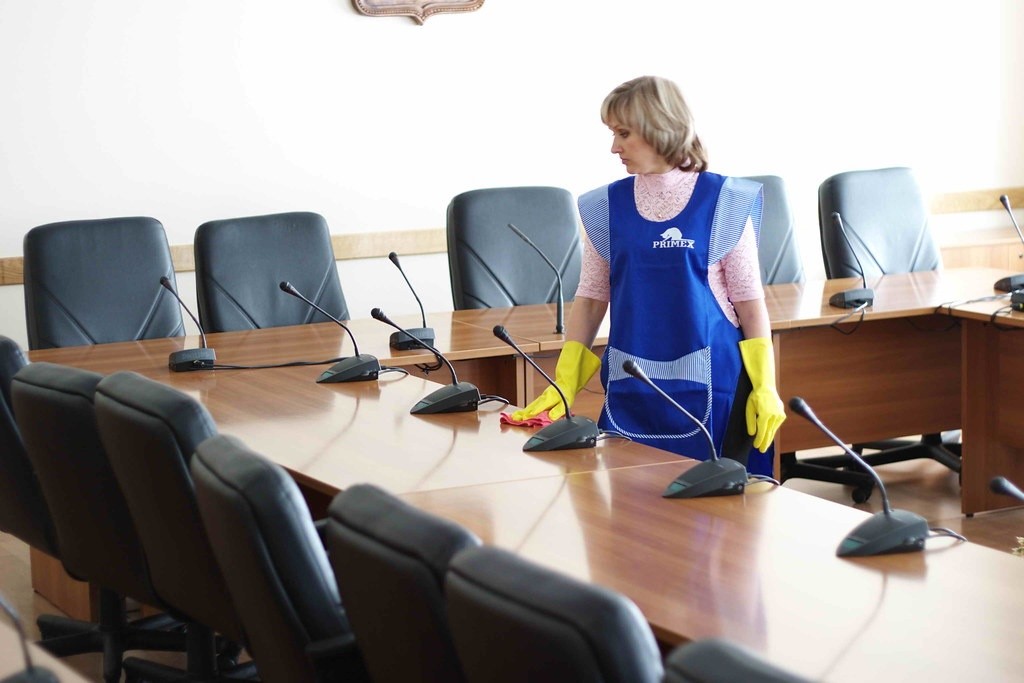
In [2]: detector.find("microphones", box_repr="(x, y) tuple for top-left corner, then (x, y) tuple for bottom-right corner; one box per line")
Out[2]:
(388, 252), (435, 351)
(788, 396), (929, 558)
(988, 474), (1024, 501)
(507, 223), (565, 333)
(829, 212), (874, 309)
(371, 307), (482, 415)
(493, 324), (599, 451)
(1000, 195), (1024, 244)
(159, 276), (215, 371)
(279, 280), (381, 384)
(622, 359), (748, 499)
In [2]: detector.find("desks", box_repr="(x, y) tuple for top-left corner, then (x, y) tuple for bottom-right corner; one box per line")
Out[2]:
(29, 268), (1024, 682)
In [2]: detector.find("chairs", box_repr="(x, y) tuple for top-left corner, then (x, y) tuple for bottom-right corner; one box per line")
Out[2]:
(0, 171), (873, 683)
(818, 165), (963, 483)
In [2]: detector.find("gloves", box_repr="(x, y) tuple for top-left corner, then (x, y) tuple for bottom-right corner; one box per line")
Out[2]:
(739, 337), (786, 453)
(511, 341), (601, 422)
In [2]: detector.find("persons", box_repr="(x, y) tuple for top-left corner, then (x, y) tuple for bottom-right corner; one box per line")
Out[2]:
(510, 76), (787, 462)
